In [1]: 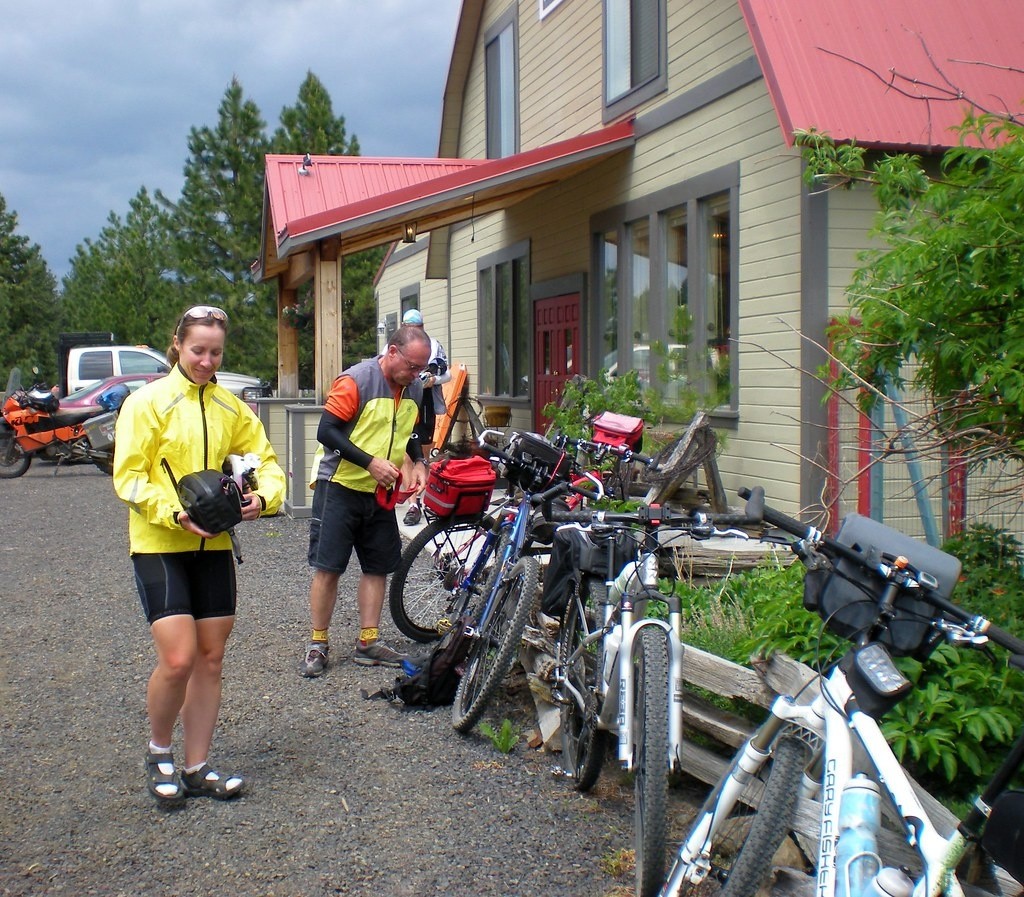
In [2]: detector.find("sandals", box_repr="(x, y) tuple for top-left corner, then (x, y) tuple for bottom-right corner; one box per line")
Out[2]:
(143, 748), (184, 799)
(180, 763), (244, 799)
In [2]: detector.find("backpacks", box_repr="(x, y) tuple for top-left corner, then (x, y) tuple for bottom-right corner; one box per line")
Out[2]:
(394, 614), (478, 709)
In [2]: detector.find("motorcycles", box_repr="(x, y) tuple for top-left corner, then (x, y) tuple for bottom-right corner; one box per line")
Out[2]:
(0, 367), (127, 479)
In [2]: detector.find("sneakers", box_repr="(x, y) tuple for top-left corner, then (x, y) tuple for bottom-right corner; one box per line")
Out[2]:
(403, 498), (421, 525)
(354, 637), (410, 667)
(300, 642), (328, 677)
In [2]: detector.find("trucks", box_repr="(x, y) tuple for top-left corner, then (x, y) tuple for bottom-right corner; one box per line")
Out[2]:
(602, 345), (720, 393)
(56, 332), (273, 420)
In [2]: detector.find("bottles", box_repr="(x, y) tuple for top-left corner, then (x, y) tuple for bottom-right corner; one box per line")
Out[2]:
(608, 561), (643, 603)
(863, 865), (915, 897)
(604, 625), (622, 681)
(833, 771), (881, 896)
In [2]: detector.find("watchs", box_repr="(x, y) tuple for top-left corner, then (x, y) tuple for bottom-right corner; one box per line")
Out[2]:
(415, 457), (428, 467)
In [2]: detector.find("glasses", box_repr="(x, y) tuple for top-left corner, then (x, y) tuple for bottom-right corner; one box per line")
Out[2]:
(396, 348), (429, 373)
(175, 306), (228, 335)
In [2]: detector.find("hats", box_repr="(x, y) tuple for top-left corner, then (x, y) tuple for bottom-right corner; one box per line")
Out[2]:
(403, 308), (423, 325)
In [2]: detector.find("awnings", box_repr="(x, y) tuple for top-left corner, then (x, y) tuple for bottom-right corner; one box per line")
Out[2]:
(743, 0), (1024, 156)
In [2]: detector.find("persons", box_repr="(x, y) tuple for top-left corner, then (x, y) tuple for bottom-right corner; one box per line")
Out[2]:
(384, 309), (451, 526)
(297, 326), (429, 678)
(112, 307), (286, 802)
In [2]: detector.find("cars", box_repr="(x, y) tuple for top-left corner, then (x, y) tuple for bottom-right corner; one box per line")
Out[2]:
(58, 373), (168, 409)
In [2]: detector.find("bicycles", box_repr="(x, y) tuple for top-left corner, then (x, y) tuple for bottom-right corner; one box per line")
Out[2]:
(390, 416), (654, 732)
(542, 483), (764, 896)
(657, 504), (1024, 897)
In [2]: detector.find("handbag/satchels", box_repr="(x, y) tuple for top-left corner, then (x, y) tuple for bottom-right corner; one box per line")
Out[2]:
(176, 469), (246, 564)
(422, 455), (496, 516)
(540, 521), (638, 617)
